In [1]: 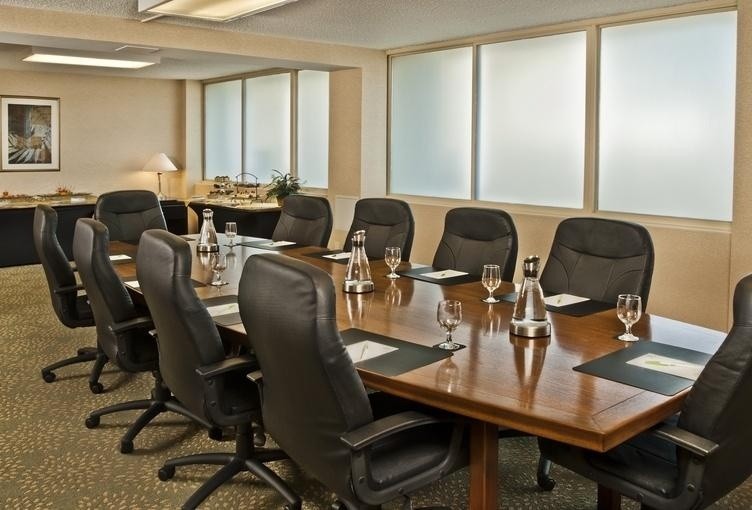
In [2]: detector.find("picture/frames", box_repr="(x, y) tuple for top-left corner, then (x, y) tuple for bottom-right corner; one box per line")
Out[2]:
(0, 96), (61, 172)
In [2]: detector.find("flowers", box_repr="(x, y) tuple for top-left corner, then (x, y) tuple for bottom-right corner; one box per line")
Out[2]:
(56, 185), (71, 197)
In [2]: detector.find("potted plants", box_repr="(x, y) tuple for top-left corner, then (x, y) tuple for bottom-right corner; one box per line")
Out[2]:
(266, 170), (302, 206)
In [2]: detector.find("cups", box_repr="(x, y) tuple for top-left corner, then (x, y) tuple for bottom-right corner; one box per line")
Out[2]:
(435, 299), (462, 351)
(224, 222), (237, 246)
(383, 246), (401, 278)
(481, 264), (501, 304)
(615, 294), (642, 342)
(209, 253), (226, 286)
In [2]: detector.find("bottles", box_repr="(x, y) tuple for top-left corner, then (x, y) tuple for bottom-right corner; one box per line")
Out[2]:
(509, 255), (551, 337)
(342, 229), (374, 294)
(197, 209), (219, 253)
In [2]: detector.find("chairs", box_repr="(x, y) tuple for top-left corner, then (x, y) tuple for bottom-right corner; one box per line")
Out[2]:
(497, 218), (654, 490)
(73, 218), (221, 452)
(431, 208), (517, 283)
(237, 254), (469, 509)
(270, 195), (331, 246)
(134, 229), (301, 509)
(95, 190), (168, 241)
(33, 204), (107, 393)
(537, 272), (752, 510)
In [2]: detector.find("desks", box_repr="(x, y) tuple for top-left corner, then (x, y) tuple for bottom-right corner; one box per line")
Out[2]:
(188, 197), (281, 238)
(109, 233), (727, 510)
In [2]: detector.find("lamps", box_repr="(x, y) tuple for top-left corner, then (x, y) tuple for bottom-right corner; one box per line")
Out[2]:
(22, 54), (155, 71)
(138, 1), (290, 28)
(143, 152), (178, 200)
